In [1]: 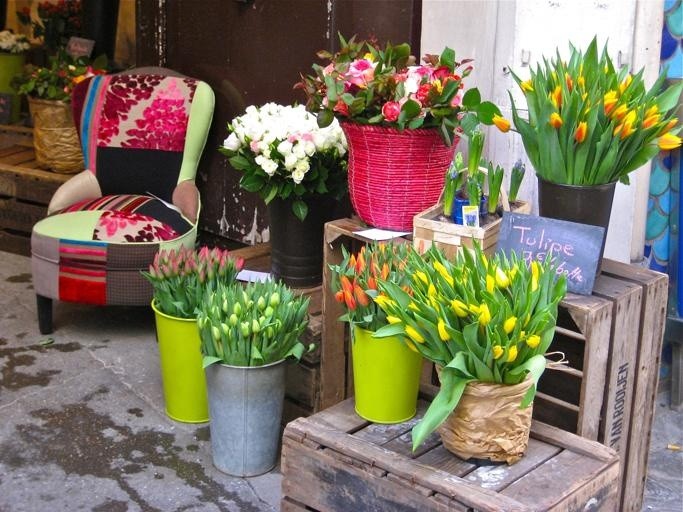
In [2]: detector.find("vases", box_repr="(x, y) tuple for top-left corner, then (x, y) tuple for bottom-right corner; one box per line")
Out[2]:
(336, 119), (460, 231)
(261, 188), (347, 288)
(25, 94), (84, 174)
(535, 173), (618, 279)
(346, 325), (423, 425)
(150, 298), (208, 423)
(414, 193), (531, 265)
(0, 53), (23, 124)
(435, 367), (538, 466)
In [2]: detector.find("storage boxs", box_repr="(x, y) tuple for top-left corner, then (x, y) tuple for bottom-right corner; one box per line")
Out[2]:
(1, 144), (82, 256)
(320, 214), (412, 410)
(278, 392), (624, 511)
(225, 242), (322, 432)
(554, 257), (669, 510)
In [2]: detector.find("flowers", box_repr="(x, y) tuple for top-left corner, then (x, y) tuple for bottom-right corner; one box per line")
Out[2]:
(0, 11), (45, 54)
(16, 54), (107, 103)
(289, 31), (501, 145)
(141, 243), (245, 318)
(221, 102), (350, 219)
(362, 234), (565, 451)
(412, 134), (528, 221)
(327, 240), (448, 344)
(492, 32), (682, 186)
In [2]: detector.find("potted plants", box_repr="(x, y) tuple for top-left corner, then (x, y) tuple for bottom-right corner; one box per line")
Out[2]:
(196, 273), (320, 479)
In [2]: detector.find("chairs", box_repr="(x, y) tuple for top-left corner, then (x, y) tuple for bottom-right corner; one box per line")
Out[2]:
(31, 64), (216, 336)
